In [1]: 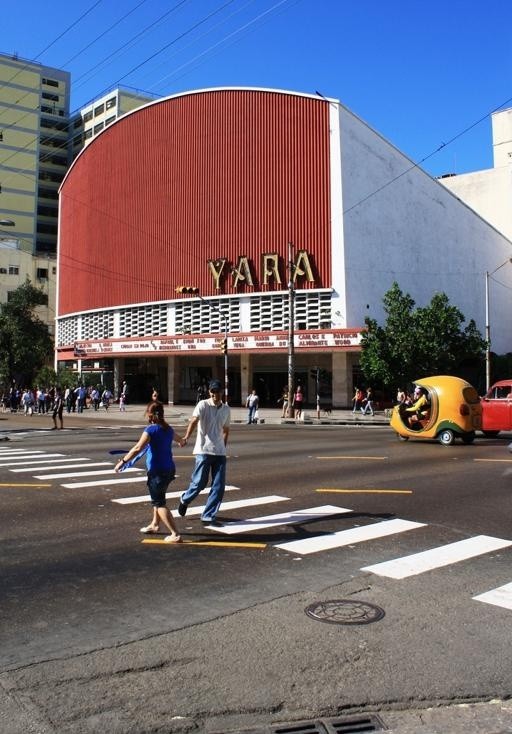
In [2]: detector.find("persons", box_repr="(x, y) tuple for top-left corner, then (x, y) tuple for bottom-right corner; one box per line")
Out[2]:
(292, 384), (305, 420)
(396, 386), (430, 430)
(361, 387), (375, 416)
(351, 383), (365, 415)
(276, 385), (288, 418)
(151, 386), (158, 401)
(0, 380), (130, 430)
(177, 378), (231, 529)
(113, 400), (185, 543)
(245, 389), (260, 424)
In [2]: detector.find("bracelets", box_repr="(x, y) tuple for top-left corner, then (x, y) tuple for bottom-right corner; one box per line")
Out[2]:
(122, 457), (127, 463)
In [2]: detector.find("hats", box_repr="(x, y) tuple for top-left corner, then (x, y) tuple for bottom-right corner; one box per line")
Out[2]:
(209, 379), (224, 392)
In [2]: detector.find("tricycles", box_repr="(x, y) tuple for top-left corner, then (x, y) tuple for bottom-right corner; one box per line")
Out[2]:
(389, 373), (484, 446)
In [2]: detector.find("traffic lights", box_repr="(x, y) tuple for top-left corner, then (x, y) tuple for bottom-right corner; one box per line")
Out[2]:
(220, 338), (227, 355)
(175, 285), (200, 296)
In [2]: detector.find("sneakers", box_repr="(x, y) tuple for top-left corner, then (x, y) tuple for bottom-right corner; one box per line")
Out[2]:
(178, 503), (188, 516)
(140, 525), (160, 532)
(201, 521), (223, 528)
(164, 535), (184, 543)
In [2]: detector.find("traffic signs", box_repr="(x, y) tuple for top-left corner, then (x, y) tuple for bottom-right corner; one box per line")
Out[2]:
(310, 367), (328, 380)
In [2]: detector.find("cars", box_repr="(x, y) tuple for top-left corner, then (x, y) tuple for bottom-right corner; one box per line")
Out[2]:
(480, 380), (512, 438)
